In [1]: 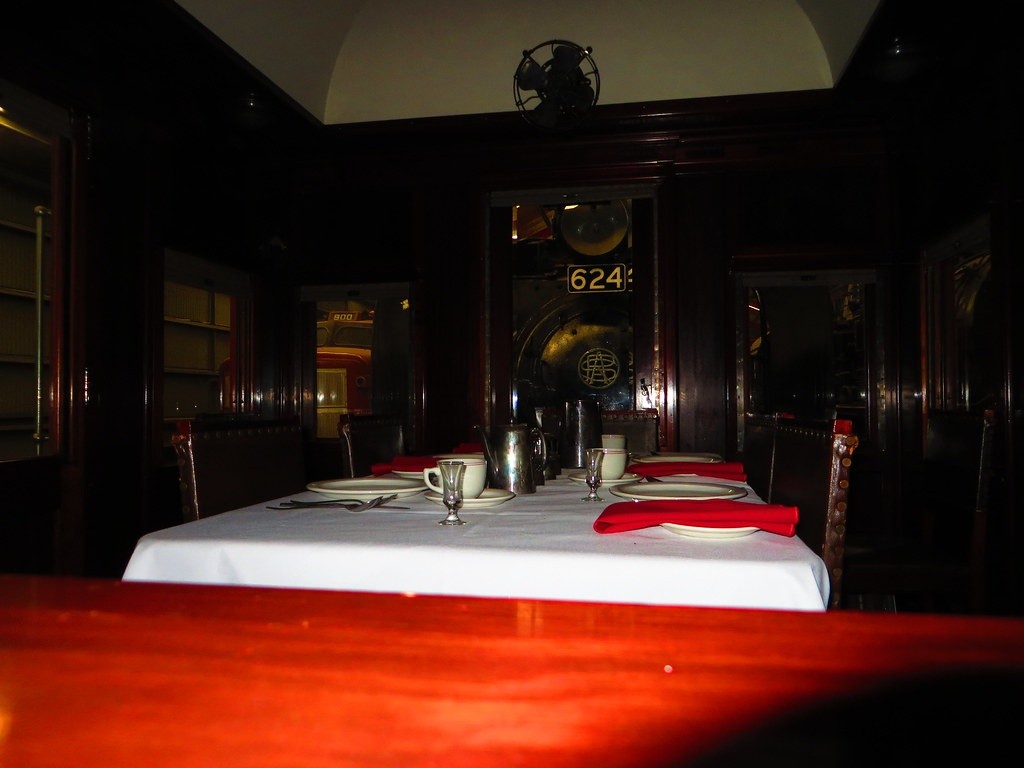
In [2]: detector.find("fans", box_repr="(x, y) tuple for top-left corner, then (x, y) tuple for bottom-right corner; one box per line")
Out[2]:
(513, 39), (600, 133)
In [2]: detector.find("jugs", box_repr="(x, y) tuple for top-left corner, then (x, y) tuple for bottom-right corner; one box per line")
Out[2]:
(472, 418), (547, 495)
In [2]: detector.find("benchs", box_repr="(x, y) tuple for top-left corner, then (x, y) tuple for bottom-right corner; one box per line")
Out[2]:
(174, 414), (308, 524)
(539, 412), (657, 452)
(740, 408), (860, 608)
(338, 413), (409, 479)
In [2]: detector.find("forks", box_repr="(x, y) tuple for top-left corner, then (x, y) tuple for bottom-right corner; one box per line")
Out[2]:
(290, 493), (398, 506)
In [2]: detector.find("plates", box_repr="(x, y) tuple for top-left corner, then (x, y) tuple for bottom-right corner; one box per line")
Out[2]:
(392, 470), (424, 479)
(660, 523), (761, 538)
(633, 454), (722, 464)
(423, 489), (515, 507)
(569, 471), (643, 486)
(432, 454), (484, 458)
(306, 477), (428, 500)
(607, 482), (747, 501)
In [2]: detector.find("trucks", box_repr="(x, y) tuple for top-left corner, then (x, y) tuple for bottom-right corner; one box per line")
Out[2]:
(222, 310), (404, 461)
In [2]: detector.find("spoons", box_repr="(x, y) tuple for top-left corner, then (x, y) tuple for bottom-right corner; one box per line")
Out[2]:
(265, 496), (383, 513)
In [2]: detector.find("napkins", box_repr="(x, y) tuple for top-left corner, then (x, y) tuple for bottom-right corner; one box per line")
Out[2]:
(371, 455), (451, 475)
(592, 499), (800, 538)
(626, 463), (748, 483)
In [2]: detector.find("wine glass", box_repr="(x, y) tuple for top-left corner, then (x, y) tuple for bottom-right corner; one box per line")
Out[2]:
(580, 448), (605, 504)
(437, 462), (467, 525)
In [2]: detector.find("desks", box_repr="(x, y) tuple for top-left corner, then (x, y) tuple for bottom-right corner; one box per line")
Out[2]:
(120, 451), (831, 613)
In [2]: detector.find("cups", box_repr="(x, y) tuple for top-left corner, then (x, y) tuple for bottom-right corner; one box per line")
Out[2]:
(594, 449), (626, 480)
(423, 459), (486, 499)
(601, 435), (626, 448)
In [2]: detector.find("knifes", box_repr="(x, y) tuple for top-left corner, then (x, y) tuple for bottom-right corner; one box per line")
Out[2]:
(279, 502), (411, 509)
